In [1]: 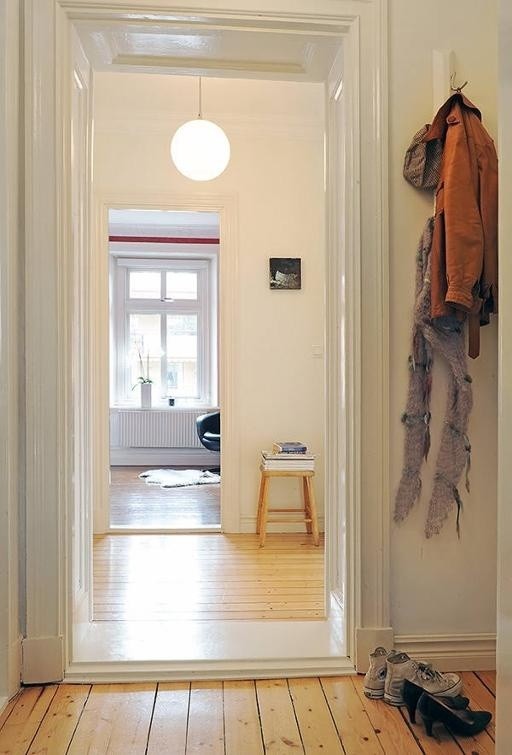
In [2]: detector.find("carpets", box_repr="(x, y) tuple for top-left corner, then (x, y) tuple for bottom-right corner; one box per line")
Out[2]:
(136, 467), (220, 488)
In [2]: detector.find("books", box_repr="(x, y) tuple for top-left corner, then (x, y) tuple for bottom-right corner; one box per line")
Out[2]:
(264, 441), (314, 470)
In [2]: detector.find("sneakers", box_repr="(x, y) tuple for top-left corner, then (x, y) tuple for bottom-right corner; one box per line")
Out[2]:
(363, 645), (462, 707)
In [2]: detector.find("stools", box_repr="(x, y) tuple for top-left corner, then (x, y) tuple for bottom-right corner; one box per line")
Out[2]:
(254, 466), (317, 549)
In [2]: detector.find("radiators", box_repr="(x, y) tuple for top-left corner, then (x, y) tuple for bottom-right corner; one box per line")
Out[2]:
(117, 409), (209, 448)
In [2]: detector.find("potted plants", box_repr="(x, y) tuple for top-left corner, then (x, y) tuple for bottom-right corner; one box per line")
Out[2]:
(134, 376), (152, 407)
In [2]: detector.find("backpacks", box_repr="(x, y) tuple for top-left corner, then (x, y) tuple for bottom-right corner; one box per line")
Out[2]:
(403, 123), (444, 189)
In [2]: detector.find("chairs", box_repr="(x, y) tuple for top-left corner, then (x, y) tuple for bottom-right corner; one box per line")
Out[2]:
(195, 413), (220, 474)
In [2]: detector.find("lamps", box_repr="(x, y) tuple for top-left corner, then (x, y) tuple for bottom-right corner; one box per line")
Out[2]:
(173, 78), (229, 183)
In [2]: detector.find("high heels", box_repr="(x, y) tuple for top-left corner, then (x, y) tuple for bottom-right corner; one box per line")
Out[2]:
(400, 677), (492, 737)
